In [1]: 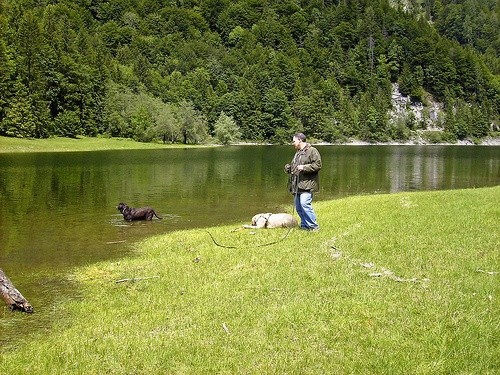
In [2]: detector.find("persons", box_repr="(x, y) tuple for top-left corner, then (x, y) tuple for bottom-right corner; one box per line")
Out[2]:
(284, 131), (324, 232)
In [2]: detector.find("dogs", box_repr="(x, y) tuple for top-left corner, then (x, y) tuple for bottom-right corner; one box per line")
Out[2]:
(115, 202), (163, 222)
(242, 213), (299, 229)
